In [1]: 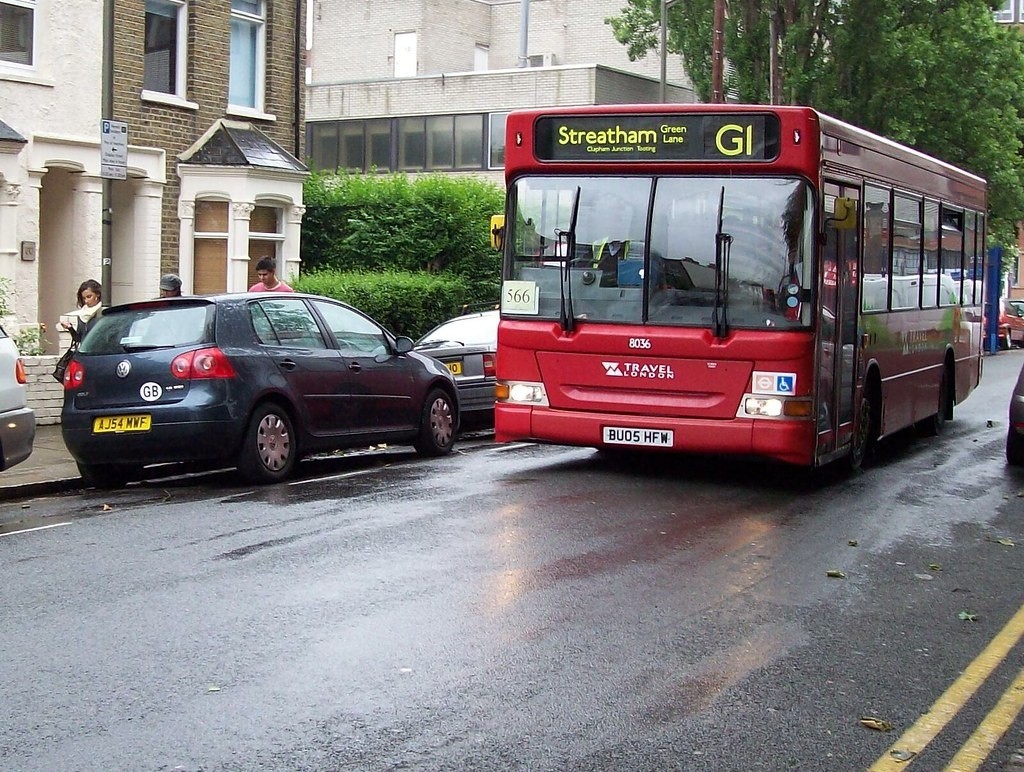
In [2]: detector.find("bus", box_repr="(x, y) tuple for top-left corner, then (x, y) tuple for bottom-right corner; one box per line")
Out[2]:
(489, 101), (989, 483)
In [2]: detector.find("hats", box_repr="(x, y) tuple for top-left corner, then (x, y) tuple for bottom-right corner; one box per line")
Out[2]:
(160, 274), (181, 291)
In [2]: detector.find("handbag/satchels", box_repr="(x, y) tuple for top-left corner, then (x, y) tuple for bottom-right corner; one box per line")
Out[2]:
(52, 343), (78, 383)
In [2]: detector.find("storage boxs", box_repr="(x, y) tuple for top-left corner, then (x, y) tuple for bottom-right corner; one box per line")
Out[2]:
(616, 259), (660, 289)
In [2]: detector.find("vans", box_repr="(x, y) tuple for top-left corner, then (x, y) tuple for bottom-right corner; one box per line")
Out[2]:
(854, 265), (986, 341)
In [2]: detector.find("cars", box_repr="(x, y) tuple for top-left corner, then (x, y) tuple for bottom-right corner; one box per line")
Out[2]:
(410, 310), (501, 433)
(0, 324), (37, 473)
(60, 291), (461, 492)
(1006, 365), (1024, 467)
(979, 296), (1024, 350)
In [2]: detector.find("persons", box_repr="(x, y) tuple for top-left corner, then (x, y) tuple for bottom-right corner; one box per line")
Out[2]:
(575, 205), (667, 292)
(159, 274), (183, 299)
(249, 257), (294, 293)
(61, 279), (108, 344)
